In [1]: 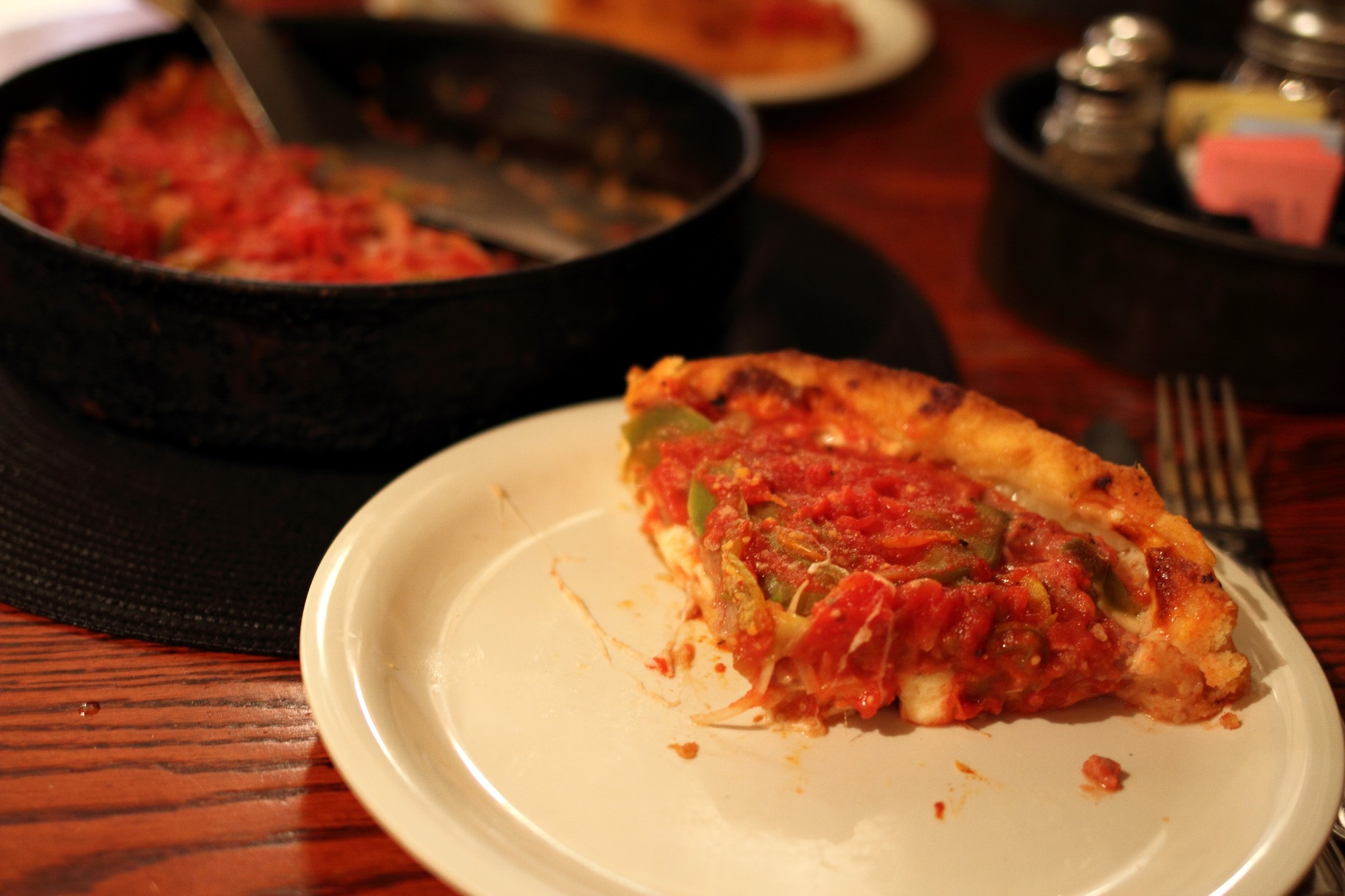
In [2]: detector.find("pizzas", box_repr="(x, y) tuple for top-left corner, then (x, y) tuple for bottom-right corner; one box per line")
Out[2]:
(609, 340), (1253, 744)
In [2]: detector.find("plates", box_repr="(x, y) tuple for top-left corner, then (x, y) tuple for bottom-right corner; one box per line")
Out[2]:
(494, 3), (923, 106)
(300, 388), (1343, 896)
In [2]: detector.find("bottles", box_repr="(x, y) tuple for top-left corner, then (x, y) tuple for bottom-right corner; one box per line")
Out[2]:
(1235, 4), (1343, 108)
(1044, 14), (1160, 187)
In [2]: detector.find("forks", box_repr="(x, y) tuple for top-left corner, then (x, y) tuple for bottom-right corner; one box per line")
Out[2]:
(1139, 370), (1336, 896)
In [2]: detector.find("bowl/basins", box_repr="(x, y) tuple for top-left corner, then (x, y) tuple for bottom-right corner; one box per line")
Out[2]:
(977, 37), (1345, 396)
(3, 17), (763, 444)
